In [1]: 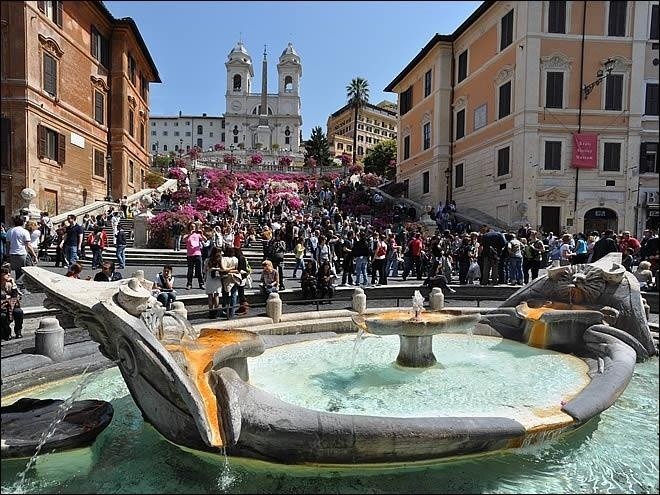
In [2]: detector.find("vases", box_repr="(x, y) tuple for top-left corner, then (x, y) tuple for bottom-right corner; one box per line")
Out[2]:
(165, 232), (182, 248)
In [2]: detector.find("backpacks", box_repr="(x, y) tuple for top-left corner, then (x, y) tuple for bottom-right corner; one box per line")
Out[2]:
(90, 233), (101, 246)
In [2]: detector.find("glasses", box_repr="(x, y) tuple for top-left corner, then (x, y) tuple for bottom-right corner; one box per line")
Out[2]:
(103, 266), (110, 270)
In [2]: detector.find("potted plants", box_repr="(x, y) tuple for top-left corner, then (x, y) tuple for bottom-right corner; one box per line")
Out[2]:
(236, 142), (280, 154)
(144, 172), (165, 189)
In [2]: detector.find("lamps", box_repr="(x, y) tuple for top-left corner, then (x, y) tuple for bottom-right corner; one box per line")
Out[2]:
(583, 57), (616, 101)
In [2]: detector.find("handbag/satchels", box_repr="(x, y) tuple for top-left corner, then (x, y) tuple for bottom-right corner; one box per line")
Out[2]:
(388, 250), (398, 260)
(0, 299), (21, 311)
(334, 241), (344, 258)
(377, 246), (387, 257)
(265, 245), (285, 262)
(560, 259), (570, 265)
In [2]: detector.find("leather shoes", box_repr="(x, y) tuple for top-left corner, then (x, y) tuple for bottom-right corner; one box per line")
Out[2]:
(4, 331), (22, 341)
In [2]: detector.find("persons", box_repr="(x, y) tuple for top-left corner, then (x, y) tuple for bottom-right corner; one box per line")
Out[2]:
(455, 221), (658, 285)
(1, 266), (23, 339)
(155, 171), (456, 316)
(1, 196), (140, 284)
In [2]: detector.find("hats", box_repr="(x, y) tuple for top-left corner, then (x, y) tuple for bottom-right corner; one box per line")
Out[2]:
(592, 228), (650, 237)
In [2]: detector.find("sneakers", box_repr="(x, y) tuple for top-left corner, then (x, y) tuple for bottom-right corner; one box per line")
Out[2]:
(341, 279), (383, 286)
(206, 301), (248, 317)
(185, 284), (205, 290)
